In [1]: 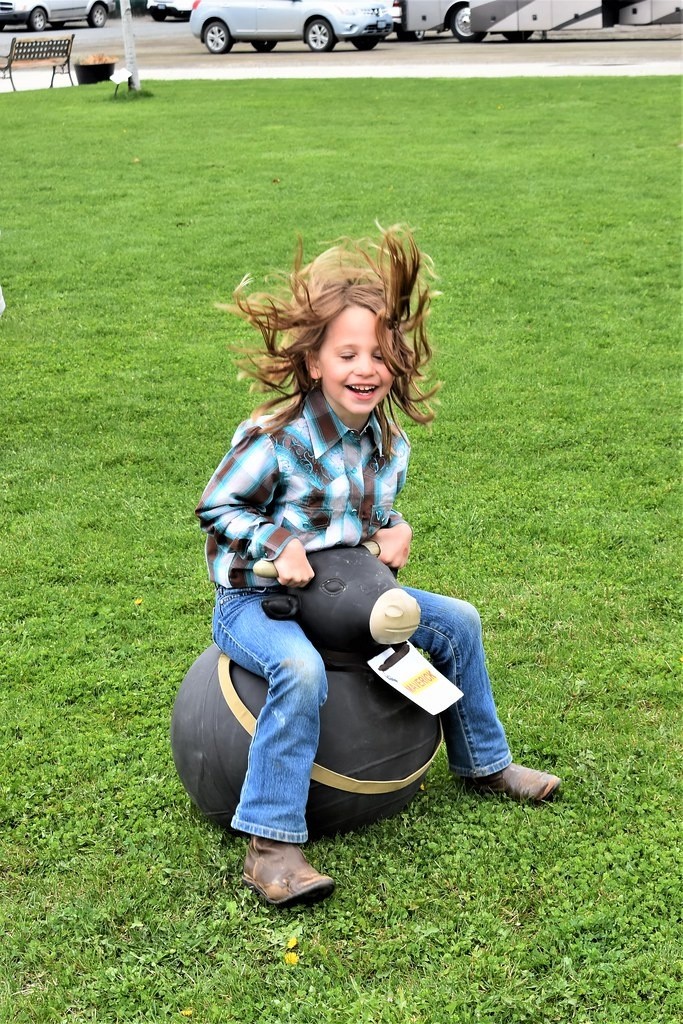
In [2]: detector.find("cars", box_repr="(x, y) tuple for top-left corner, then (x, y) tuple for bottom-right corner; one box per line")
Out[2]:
(390, 0), (425, 41)
(147, 0), (194, 22)
(0, 0), (116, 32)
(188, 0), (393, 55)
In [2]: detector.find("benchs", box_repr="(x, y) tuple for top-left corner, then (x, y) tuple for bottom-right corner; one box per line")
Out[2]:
(1, 34), (74, 91)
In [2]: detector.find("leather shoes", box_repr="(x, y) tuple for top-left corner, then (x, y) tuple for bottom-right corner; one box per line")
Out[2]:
(242, 835), (335, 906)
(460, 763), (562, 800)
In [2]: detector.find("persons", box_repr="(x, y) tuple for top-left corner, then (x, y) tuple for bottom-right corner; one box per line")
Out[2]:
(194, 222), (560, 903)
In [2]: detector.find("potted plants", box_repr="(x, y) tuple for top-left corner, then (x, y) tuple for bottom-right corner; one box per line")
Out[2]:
(73, 53), (118, 84)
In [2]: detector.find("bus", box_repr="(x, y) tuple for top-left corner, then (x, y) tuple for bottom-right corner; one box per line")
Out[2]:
(404, 0), (535, 43)
